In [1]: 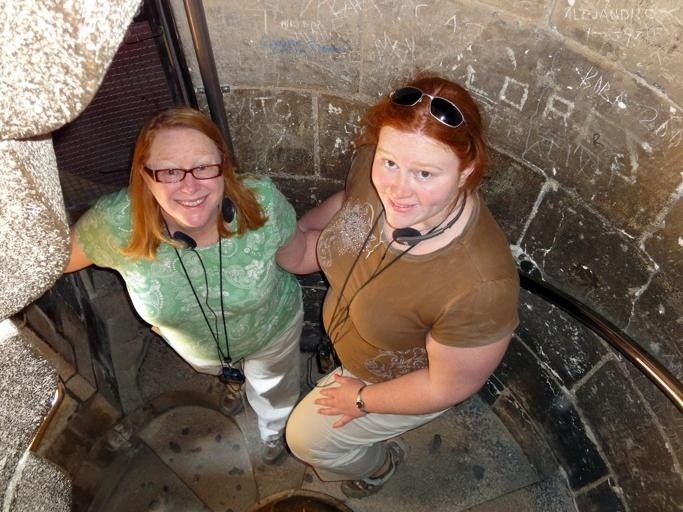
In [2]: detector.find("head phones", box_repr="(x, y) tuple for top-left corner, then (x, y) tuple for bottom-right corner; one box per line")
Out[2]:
(159, 197), (236, 251)
(391, 186), (468, 246)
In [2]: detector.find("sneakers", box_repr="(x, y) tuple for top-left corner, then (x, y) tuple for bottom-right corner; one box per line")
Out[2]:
(261, 433), (291, 467)
(341, 438), (406, 498)
(218, 357), (245, 415)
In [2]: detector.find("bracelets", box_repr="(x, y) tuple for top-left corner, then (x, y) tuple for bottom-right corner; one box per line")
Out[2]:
(354, 384), (371, 414)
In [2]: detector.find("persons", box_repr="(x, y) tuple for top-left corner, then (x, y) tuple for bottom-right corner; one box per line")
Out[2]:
(271, 76), (521, 502)
(54, 104), (307, 470)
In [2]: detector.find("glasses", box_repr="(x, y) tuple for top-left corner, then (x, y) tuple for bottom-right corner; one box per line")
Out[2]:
(383, 87), (472, 136)
(142, 159), (226, 184)
